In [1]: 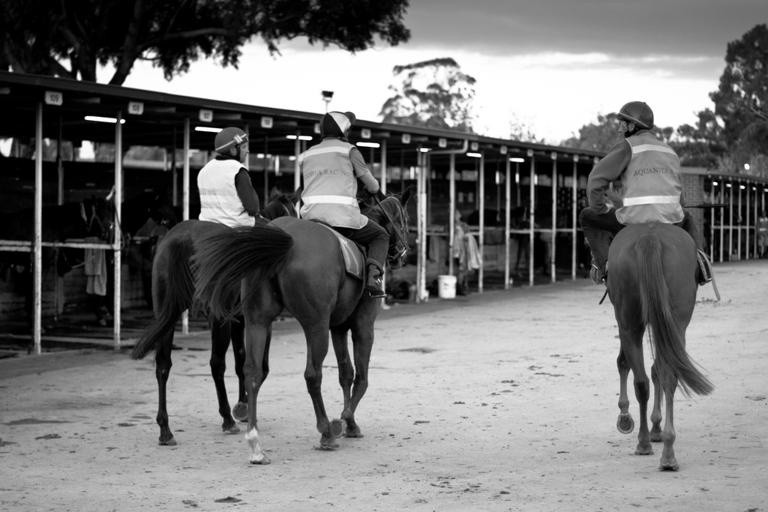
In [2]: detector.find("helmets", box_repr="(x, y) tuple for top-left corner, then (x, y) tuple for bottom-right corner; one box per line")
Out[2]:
(214, 126), (249, 154)
(616, 101), (654, 129)
(320, 111), (356, 138)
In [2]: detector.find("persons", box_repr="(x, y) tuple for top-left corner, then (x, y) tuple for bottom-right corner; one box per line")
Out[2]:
(579, 102), (712, 285)
(300, 112), (389, 295)
(197, 127), (271, 229)
(757, 211), (768, 257)
(454, 209), (470, 295)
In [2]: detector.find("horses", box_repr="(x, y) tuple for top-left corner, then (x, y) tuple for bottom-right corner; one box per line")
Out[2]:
(131, 185), (305, 445)
(191, 186), (416, 465)
(607, 222), (717, 471)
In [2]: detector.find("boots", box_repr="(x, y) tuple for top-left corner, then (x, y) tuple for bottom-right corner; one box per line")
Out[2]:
(367, 236), (390, 297)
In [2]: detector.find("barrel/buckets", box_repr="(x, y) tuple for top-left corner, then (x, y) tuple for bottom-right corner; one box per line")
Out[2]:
(438, 275), (457, 299)
